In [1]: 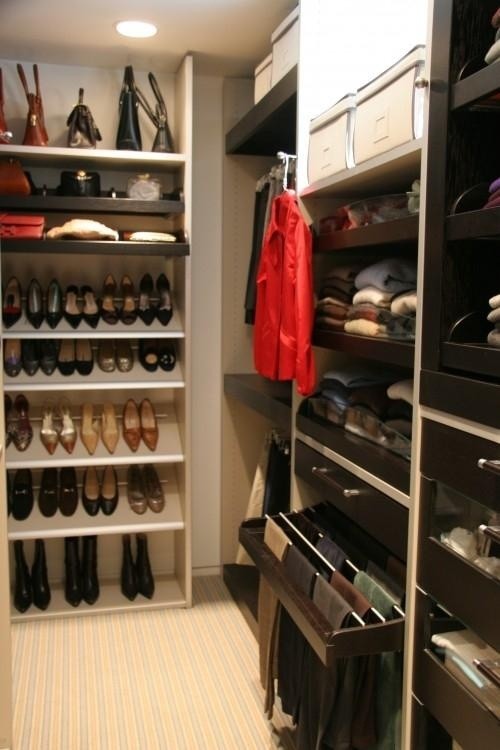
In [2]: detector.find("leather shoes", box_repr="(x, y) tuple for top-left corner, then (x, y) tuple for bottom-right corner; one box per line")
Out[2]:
(4, 339), (177, 376)
(7, 463), (165, 520)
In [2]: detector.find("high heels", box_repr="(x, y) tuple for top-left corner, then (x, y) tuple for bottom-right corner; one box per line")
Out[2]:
(5, 394), (157, 455)
(2, 273), (172, 329)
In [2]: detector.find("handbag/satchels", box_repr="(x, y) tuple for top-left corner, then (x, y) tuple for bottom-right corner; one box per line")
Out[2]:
(0, 64), (173, 152)
(0, 214), (175, 242)
(0, 158), (161, 199)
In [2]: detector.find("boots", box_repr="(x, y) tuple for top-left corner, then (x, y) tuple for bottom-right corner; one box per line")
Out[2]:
(14, 533), (153, 613)
